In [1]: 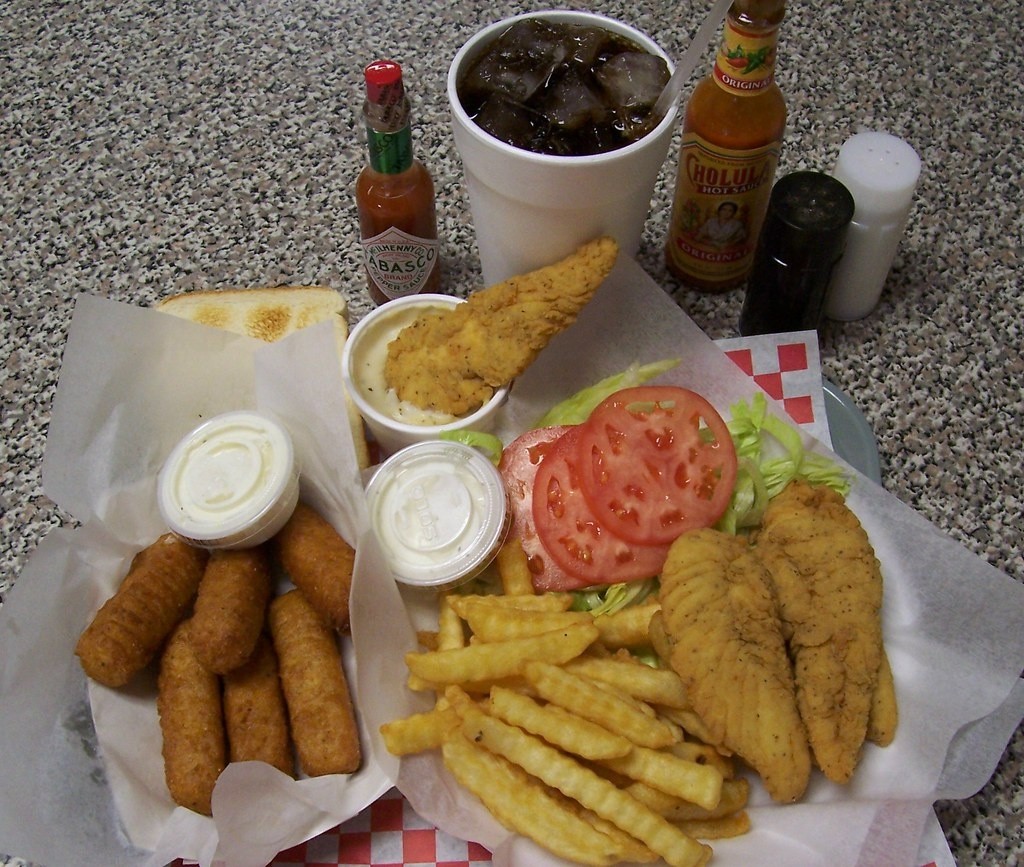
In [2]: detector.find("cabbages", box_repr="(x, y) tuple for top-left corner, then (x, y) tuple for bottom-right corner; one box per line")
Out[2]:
(441, 356), (853, 668)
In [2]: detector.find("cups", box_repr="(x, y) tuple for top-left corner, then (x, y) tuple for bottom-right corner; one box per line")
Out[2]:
(342, 291), (514, 455)
(446, 10), (679, 289)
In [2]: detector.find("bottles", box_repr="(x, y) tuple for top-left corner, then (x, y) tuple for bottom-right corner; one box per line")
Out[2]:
(739, 172), (855, 336)
(664, 0), (787, 296)
(822, 132), (921, 321)
(354, 60), (444, 306)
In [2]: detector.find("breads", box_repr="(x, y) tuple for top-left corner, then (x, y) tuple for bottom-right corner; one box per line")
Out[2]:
(148, 285), (368, 471)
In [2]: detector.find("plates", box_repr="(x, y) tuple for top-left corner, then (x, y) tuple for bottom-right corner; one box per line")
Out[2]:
(821, 376), (881, 487)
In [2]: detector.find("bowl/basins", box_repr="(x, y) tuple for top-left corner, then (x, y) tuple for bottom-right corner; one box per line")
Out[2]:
(155, 410), (299, 550)
(365, 438), (512, 589)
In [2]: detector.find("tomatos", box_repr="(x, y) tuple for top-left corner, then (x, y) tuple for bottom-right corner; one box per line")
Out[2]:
(576, 384), (737, 544)
(498, 423), (598, 590)
(533, 423), (670, 582)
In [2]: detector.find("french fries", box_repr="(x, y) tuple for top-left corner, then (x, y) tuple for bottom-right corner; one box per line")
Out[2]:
(377, 539), (751, 867)
